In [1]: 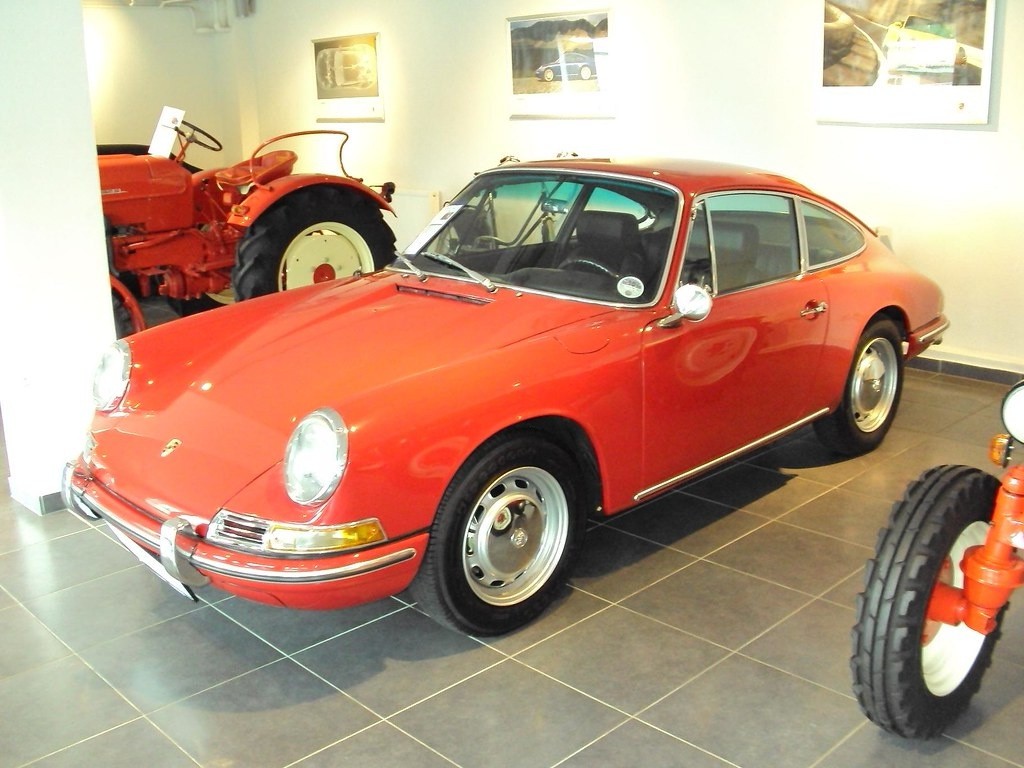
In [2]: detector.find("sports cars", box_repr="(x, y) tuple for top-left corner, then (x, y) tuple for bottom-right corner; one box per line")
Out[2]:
(59, 157), (952, 636)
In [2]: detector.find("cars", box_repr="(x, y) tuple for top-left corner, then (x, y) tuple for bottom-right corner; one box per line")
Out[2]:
(537, 53), (596, 83)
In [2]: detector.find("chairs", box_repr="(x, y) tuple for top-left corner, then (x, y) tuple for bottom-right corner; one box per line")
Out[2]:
(562, 211), (639, 273)
(639, 221), (758, 304)
(215, 150), (298, 195)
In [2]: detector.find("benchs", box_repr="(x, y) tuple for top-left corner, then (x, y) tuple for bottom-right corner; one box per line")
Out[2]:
(641, 233), (841, 283)
